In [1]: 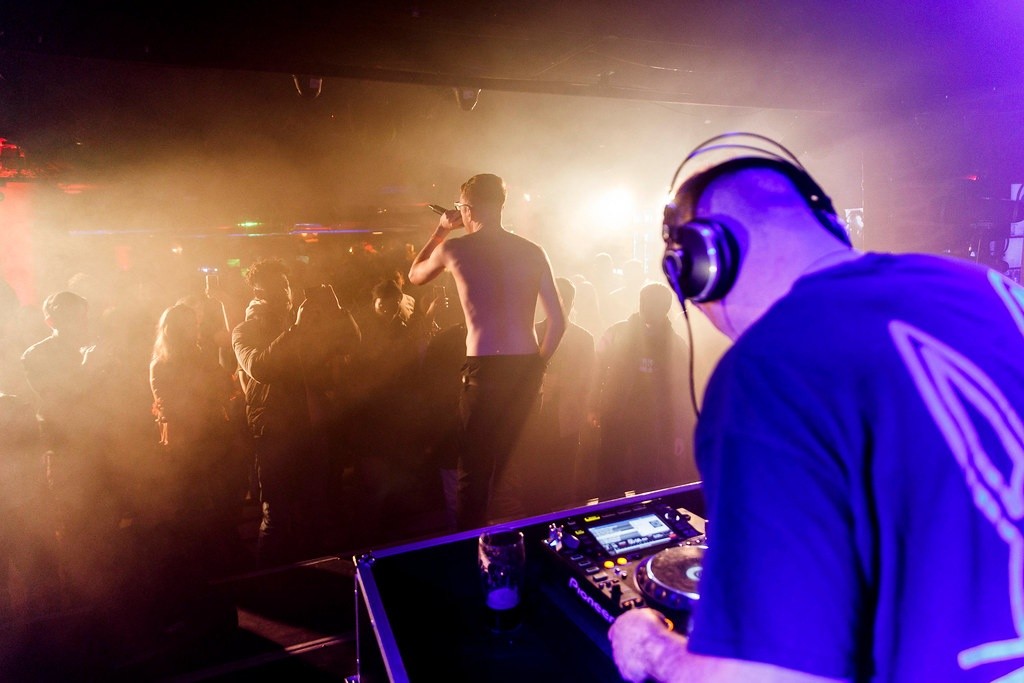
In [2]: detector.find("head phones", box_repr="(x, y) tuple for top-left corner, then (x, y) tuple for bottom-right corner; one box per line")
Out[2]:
(662, 132), (853, 304)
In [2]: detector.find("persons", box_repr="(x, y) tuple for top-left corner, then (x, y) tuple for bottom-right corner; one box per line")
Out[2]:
(606, 156), (1024, 683)
(21, 205), (688, 613)
(405, 172), (568, 535)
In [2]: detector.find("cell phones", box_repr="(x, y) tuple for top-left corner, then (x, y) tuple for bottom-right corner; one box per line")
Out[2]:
(304, 286), (325, 304)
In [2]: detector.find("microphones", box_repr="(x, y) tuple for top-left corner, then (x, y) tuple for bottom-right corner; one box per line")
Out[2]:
(429, 205), (464, 227)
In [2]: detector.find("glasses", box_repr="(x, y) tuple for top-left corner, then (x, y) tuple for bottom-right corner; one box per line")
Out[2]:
(454, 200), (470, 211)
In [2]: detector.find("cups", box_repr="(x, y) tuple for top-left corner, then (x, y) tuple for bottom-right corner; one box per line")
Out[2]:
(478, 527), (525, 610)
